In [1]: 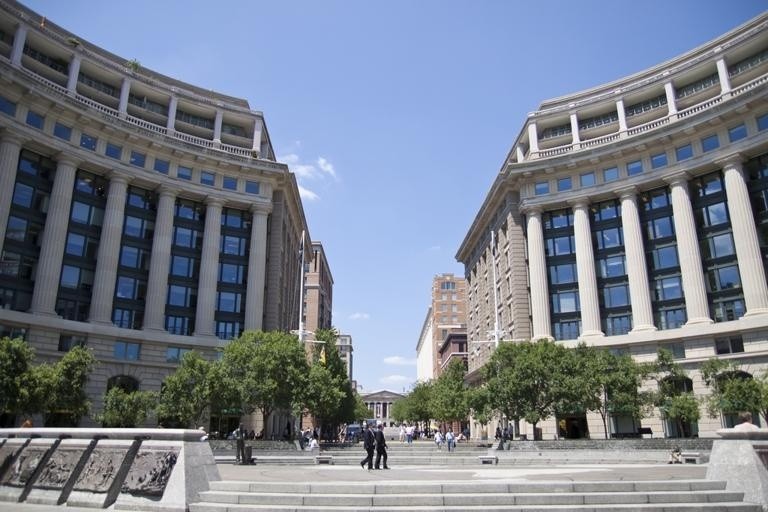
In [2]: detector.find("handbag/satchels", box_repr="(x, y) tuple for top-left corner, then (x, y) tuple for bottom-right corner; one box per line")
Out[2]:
(454, 441), (456, 447)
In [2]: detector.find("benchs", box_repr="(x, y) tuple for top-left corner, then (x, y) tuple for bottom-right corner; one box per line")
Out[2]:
(314, 455), (333, 465)
(681, 452), (701, 465)
(478, 456), (498, 465)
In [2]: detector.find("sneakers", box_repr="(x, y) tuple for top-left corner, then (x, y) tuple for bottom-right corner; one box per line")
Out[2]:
(360, 461), (391, 470)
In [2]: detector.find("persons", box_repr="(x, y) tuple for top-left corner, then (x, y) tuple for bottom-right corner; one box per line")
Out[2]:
(231, 420), (513, 471)
(667, 445), (682, 464)
(733, 410), (759, 430)
